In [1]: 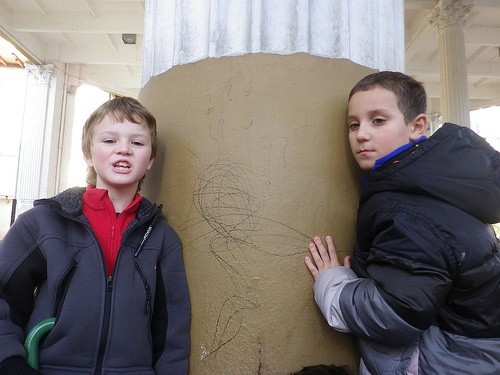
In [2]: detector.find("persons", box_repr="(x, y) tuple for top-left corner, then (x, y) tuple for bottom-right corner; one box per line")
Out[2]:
(0, 97), (191, 375)
(303, 71), (500, 375)
